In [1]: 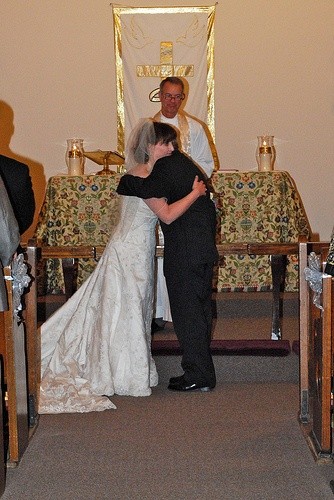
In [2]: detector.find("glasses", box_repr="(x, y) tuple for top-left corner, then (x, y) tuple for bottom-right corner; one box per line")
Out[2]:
(162, 93), (182, 101)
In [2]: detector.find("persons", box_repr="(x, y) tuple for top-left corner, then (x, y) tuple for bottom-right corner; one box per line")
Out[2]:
(89, 121), (216, 412)
(0, 174), (21, 311)
(151, 76), (215, 333)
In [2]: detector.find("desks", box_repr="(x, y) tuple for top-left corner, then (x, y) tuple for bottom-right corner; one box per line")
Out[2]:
(38, 173), (310, 296)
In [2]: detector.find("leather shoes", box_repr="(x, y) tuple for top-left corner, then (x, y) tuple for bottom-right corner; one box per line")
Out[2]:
(169, 373), (186, 383)
(168, 382), (209, 392)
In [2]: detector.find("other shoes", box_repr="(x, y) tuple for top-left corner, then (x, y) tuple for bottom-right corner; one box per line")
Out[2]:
(152, 322), (166, 331)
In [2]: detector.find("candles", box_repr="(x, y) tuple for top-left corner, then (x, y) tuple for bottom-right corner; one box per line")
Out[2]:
(259, 141), (271, 172)
(69, 144), (81, 175)
